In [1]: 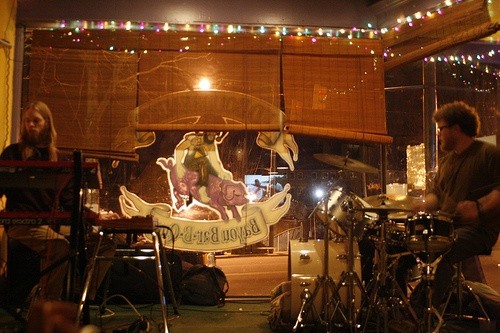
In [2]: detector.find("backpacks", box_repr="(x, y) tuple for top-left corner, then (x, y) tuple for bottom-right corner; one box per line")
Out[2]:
(179, 264), (228, 308)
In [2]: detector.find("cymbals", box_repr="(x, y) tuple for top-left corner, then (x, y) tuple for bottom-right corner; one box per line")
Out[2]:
(362, 193), (424, 219)
(313, 153), (379, 174)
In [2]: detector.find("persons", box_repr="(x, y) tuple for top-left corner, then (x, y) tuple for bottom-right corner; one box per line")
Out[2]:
(0, 102), (118, 310)
(386, 101), (500, 310)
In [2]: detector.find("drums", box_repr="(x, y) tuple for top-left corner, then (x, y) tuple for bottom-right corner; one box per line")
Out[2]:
(406, 215), (454, 252)
(312, 185), (365, 238)
(287, 238), (362, 326)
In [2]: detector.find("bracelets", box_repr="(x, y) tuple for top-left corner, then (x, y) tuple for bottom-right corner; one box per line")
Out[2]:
(472, 199), (482, 216)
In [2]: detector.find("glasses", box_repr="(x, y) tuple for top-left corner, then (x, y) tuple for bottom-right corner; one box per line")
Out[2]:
(437, 123), (457, 134)
